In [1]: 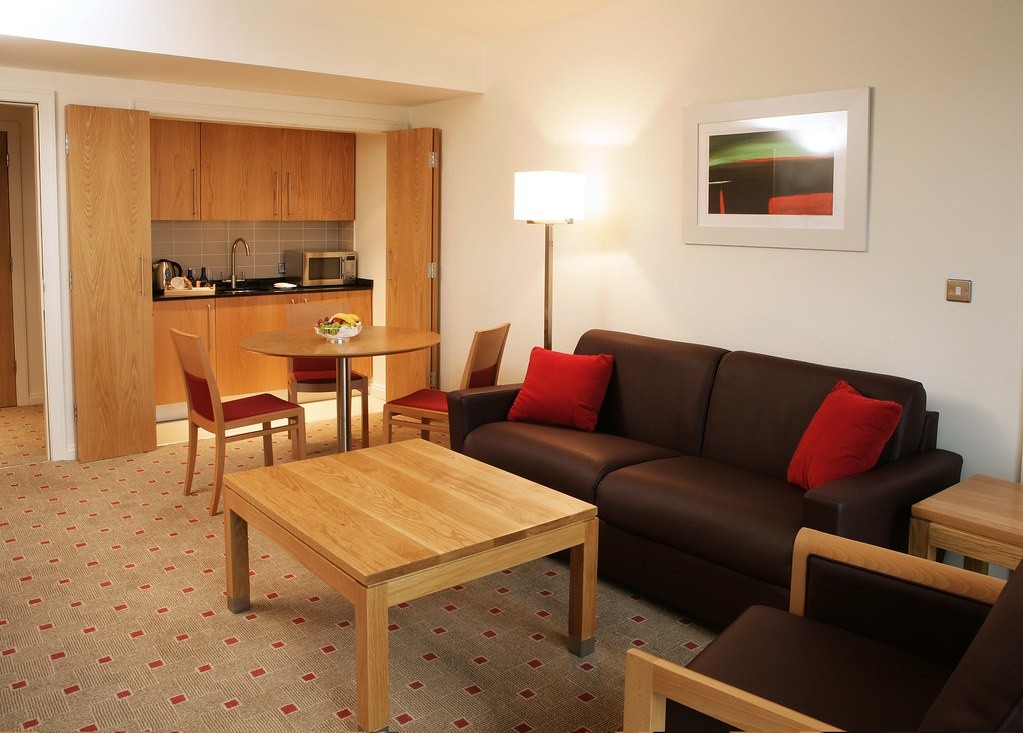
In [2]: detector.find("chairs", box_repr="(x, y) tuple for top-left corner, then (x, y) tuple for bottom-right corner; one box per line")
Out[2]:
(383, 322), (513, 445)
(282, 299), (371, 450)
(166, 327), (310, 520)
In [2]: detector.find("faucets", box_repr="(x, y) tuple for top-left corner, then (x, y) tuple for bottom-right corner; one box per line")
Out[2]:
(230, 237), (250, 289)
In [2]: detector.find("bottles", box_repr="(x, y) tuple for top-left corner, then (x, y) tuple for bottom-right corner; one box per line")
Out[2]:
(199, 267), (209, 287)
(187, 270), (196, 287)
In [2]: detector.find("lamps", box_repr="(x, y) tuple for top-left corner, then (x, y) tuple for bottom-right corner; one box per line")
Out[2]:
(509, 167), (593, 348)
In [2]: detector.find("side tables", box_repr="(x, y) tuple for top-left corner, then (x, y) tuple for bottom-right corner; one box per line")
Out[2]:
(909, 473), (1023, 572)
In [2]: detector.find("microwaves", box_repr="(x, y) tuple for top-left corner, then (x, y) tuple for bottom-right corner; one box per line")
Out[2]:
(284, 251), (357, 287)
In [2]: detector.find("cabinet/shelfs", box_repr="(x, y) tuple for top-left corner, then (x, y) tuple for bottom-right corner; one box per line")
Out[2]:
(151, 118), (355, 223)
(153, 289), (378, 426)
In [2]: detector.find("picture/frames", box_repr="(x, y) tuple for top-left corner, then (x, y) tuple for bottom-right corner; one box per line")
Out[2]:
(679, 81), (876, 254)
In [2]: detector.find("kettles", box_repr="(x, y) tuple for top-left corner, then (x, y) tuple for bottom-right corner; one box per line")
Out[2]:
(152, 258), (182, 295)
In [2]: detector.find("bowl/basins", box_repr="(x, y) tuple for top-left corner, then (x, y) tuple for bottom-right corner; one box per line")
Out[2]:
(314, 324), (362, 344)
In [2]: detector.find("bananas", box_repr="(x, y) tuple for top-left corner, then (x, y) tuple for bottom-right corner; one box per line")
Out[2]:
(329, 313), (360, 328)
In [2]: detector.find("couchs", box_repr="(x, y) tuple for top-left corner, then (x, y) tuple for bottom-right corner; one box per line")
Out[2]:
(440, 325), (966, 633)
(622, 526), (1023, 733)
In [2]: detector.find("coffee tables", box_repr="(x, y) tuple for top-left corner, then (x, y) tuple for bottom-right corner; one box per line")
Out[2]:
(212, 447), (603, 733)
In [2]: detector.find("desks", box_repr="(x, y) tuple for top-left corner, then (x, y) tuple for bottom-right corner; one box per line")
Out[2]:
(236, 322), (443, 455)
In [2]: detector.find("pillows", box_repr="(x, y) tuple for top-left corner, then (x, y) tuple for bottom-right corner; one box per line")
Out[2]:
(508, 345), (610, 433)
(781, 381), (903, 486)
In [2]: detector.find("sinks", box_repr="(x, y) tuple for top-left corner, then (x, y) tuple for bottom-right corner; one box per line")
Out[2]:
(216, 287), (271, 294)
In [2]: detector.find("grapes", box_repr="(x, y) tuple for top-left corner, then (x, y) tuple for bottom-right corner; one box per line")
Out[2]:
(318, 317), (343, 335)
